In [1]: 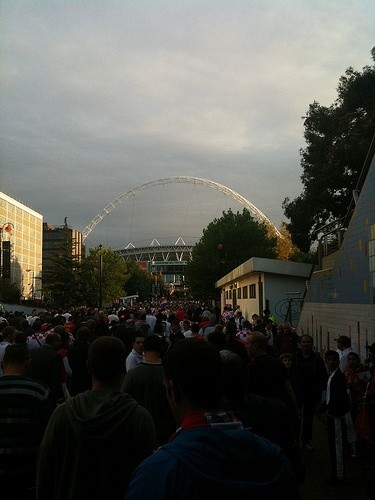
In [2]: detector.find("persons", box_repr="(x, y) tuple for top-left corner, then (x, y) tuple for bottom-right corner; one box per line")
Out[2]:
(0, 300), (375, 500)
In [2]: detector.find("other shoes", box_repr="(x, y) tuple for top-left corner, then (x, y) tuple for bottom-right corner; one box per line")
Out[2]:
(324, 477), (345, 487)
(302, 444), (315, 452)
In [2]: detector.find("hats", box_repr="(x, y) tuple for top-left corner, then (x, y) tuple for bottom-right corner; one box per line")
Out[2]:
(365, 342), (375, 350)
(333, 335), (349, 345)
